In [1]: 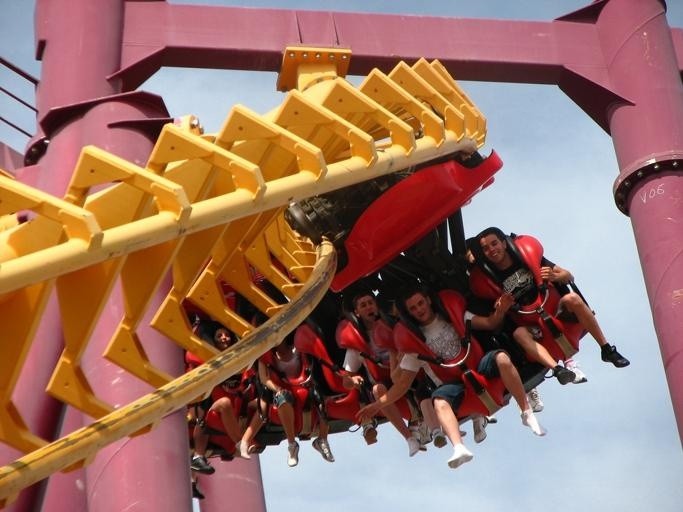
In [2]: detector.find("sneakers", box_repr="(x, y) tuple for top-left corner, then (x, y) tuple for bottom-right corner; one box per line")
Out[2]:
(363, 422), (378, 445)
(191, 452), (215, 474)
(287, 441), (299, 467)
(406, 415), (488, 468)
(602, 344), (630, 367)
(521, 358), (587, 435)
(312, 437), (335, 462)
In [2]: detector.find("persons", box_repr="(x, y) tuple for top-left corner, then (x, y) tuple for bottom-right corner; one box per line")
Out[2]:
(463, 228), (630, 386)
(190, 312), (334, 498)
(344, 283), (548, 468)
